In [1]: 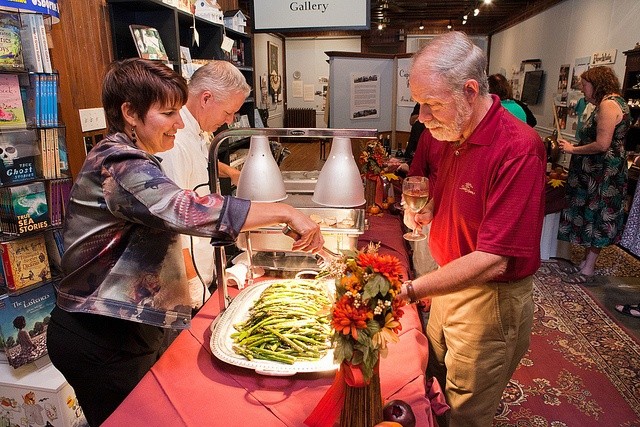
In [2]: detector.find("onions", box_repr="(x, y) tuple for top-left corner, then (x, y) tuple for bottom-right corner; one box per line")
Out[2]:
(381, 399), (416, 426)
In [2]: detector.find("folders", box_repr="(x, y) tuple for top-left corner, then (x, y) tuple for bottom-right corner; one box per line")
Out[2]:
(286, 108), (316, 142)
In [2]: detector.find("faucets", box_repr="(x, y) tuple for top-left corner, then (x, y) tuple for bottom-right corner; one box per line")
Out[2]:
(561, 264), (582, 273)
(616, 302), (639, 317)
(560, 275), (594, 284)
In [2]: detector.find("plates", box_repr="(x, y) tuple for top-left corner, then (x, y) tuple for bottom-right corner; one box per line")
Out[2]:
(0, 0), (76, 359)
(105, 0), (257, 194)
(617, 51), (640, 179)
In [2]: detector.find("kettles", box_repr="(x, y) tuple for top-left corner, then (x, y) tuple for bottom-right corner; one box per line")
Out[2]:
(231, 280), (332, 364)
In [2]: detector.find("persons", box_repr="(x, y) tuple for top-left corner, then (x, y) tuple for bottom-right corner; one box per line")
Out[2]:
(556, 65), (629, 282)
(11, 314), (37, 362)
(616, 177), (640, 317)
(46, 59), (324, 427)
(405, 102), (426, 154)
(392, 32), (547, 427)
(154, 61), (252, 310)
(486, 74), (537, 128)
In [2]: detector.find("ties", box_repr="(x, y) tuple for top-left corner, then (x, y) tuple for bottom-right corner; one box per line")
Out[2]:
(522, 71), (542, 102)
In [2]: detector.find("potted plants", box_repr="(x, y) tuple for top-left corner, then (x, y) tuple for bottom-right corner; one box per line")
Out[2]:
(267, 40), (278, 94)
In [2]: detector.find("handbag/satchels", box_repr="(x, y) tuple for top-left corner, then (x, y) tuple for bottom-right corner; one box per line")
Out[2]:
(406, 282), (418, 303)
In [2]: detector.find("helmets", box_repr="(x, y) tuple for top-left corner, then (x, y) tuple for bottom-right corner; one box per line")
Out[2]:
(207, 272), (340, 376)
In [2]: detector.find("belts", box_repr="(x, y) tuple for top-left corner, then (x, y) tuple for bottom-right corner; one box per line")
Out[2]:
(403, 176), (430, 242)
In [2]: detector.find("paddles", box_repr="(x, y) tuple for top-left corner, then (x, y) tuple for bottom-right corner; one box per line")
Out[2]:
(490, 260), (640, 427)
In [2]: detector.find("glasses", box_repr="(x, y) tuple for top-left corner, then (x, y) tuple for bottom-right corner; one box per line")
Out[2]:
(312, 137), (368, 208)
(235, 135), (289, 205)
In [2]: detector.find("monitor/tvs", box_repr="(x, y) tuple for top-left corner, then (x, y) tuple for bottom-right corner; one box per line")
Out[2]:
(0, 182), (49, 236)
(46, 229), (64, 273)
(1, 283), (58, 370)
(0, 127), (68, 185)
(0, 72), (58, 129)
(0, 12), (53, 72)
(48, 179), (72, 227)
(131, 23), (169, 63)
(0, 237), (52, 297)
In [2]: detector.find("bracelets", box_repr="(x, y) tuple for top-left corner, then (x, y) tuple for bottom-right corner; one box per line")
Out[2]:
(237, 208), (365, 260)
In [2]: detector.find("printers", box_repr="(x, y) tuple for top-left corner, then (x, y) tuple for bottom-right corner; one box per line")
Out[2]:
(281, 225), (342, 271)
